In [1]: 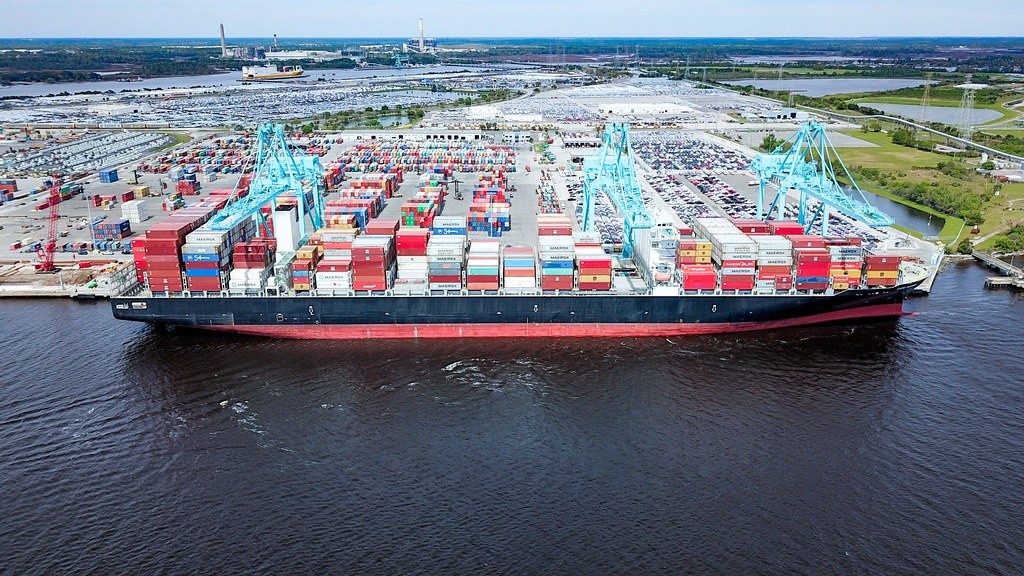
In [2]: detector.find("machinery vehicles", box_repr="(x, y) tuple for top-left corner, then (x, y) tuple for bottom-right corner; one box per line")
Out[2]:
(32, 169), (63, 273)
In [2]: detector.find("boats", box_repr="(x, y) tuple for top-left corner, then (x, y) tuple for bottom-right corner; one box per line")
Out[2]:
(243, 64), (311, 80)
